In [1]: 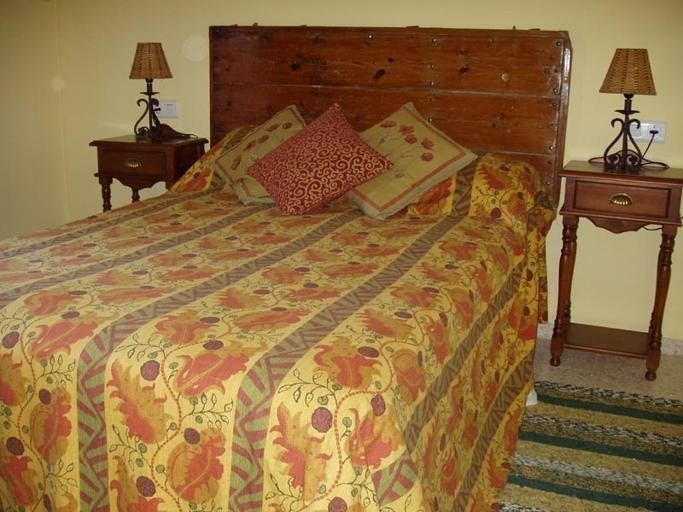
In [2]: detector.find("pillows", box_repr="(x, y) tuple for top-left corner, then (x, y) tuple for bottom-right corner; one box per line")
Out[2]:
(346, 99), (477, 222)
(208, 102), (309, 207)
(247, 102), (393, 215)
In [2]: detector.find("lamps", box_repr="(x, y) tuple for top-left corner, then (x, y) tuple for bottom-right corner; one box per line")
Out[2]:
(598, 47), (656, 172)
(129, 42), (173, 142)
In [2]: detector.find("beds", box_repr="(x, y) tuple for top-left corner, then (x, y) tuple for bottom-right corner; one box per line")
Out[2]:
(1, 25), (573, 511)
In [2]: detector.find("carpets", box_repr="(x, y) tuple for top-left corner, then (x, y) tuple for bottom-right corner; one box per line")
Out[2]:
(504, 383), (682, 512)
(629, 122), (666, 143)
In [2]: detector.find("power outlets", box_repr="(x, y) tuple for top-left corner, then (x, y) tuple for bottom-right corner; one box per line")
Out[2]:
(152, 100), (176, 119)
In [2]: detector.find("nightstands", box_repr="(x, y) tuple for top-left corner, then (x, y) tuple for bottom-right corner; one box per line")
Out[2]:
(550, 160), (682, 382)
(89, 136), (209, 212)
